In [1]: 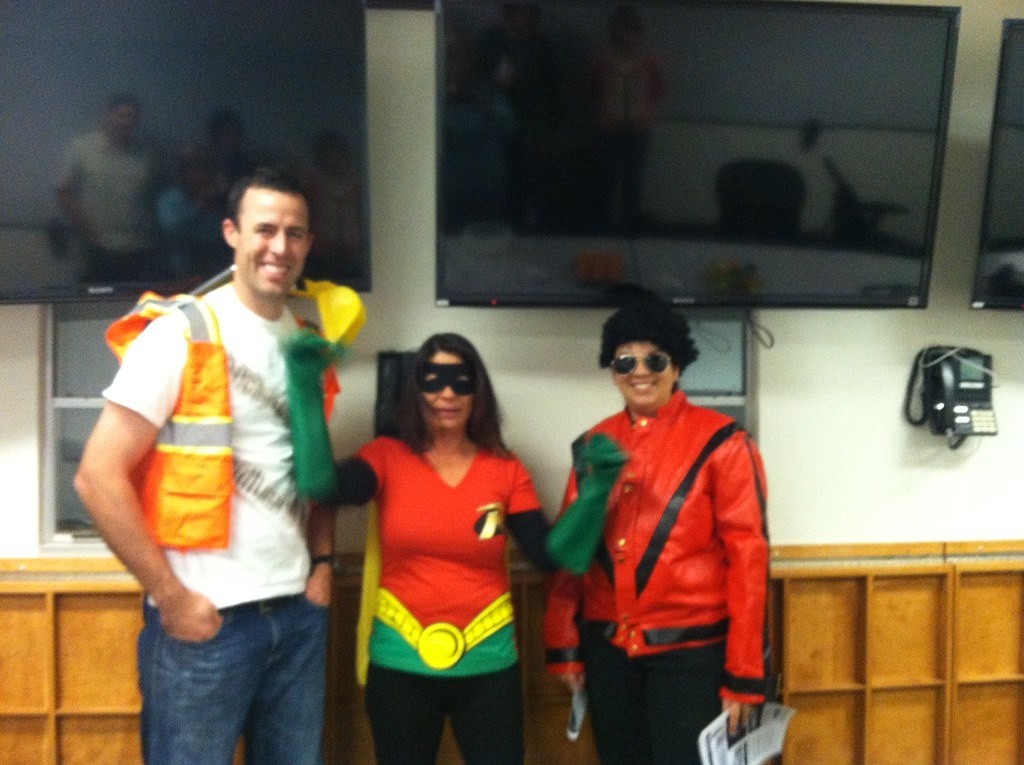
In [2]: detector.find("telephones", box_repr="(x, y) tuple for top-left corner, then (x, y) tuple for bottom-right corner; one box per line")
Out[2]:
(915, 346), (1002, 437)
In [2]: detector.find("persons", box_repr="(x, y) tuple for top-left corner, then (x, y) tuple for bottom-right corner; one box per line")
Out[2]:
(542, 296), (772, 765)
(279, 329), (624, 765)
(73, 166), (343, 765)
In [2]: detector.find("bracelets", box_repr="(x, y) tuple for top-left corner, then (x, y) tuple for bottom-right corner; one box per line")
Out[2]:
(309, 554), (335, 572)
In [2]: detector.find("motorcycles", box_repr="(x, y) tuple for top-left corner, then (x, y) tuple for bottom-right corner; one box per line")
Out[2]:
(693, 113), (913, 241)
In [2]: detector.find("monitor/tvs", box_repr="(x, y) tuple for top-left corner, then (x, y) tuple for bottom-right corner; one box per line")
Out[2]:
(972, 18), (1024, 310)
(0, 0), (373, 293)
(434, 1), (961, 312)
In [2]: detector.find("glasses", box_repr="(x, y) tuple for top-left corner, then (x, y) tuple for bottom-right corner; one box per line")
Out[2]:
(610, 353), (670, 374)
